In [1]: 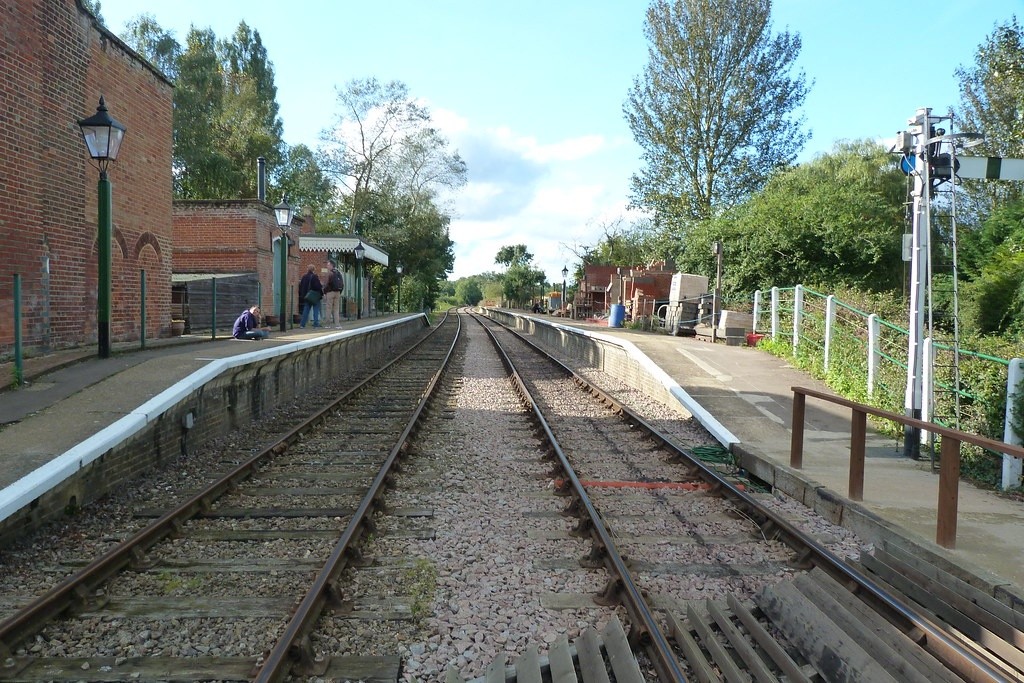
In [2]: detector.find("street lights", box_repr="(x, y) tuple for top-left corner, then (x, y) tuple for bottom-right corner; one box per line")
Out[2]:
(617, 267), (623, 305)
(711, 240), (723, 344)
(354, 240), (366, 320)
(396, 261), (402, 313)
(561, 265), (569, 317)
(78, 93), (126, 361)
(274, 192), (296, 332)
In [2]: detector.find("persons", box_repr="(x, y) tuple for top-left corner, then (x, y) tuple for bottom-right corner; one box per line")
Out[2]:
(323, 260), (344, 329)
(297, 265), (323, 328)
(534, 304), (538, 313)
(233, 306), (271, 341)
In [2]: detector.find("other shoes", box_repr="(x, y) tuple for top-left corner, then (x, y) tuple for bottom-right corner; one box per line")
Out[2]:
(336, 325), (342, 329)
(314, 325), (322, 329)
(325, 326), (330, 329)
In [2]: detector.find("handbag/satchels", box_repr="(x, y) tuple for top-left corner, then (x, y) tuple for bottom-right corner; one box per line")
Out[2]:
(305, 290), (321, 304)
